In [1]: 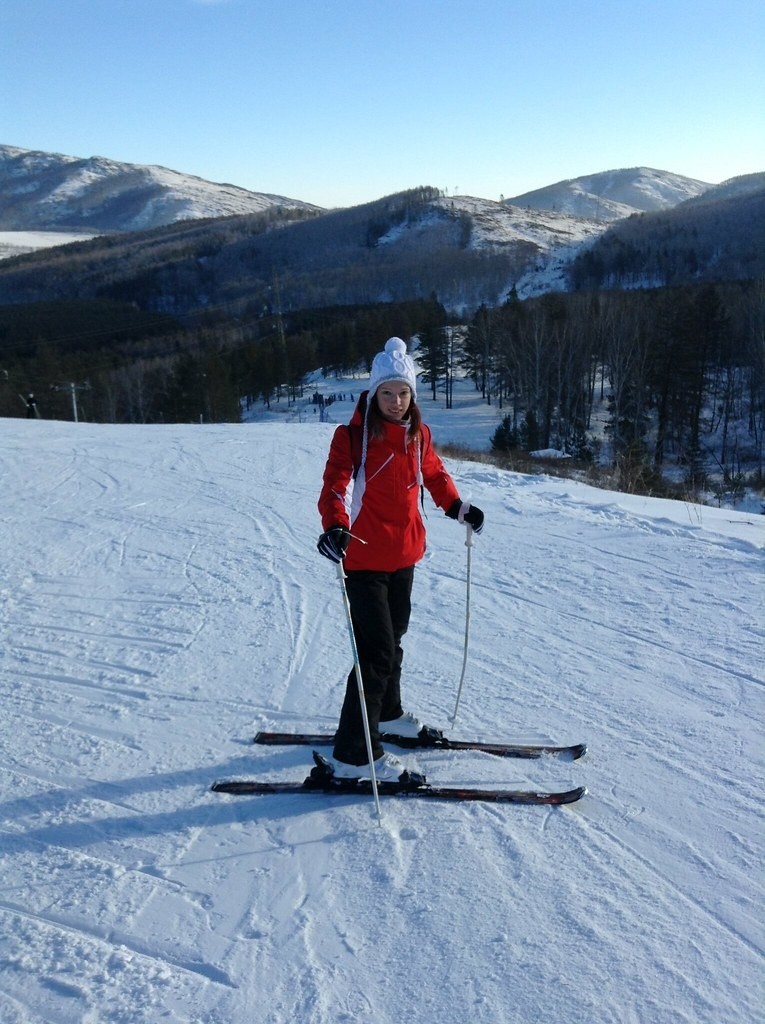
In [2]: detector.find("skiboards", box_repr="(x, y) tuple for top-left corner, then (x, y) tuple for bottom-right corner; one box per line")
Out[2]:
(211, 729), (591, 806)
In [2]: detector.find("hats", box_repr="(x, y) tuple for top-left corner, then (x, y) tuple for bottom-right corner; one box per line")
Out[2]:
(357, 336), (423, 486)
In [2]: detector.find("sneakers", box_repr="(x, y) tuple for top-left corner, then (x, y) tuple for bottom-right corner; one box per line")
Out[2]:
(331, 750), (410, 784)
(375, 709), (440, 744)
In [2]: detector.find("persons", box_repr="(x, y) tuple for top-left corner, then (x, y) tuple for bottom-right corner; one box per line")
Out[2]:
(318, 391), (354, 404)
(317, 338), (484, 780)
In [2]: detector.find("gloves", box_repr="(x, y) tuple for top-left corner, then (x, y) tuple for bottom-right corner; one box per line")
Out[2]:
(317, 525), (350, 563)
(443, 497), (484, 535)
